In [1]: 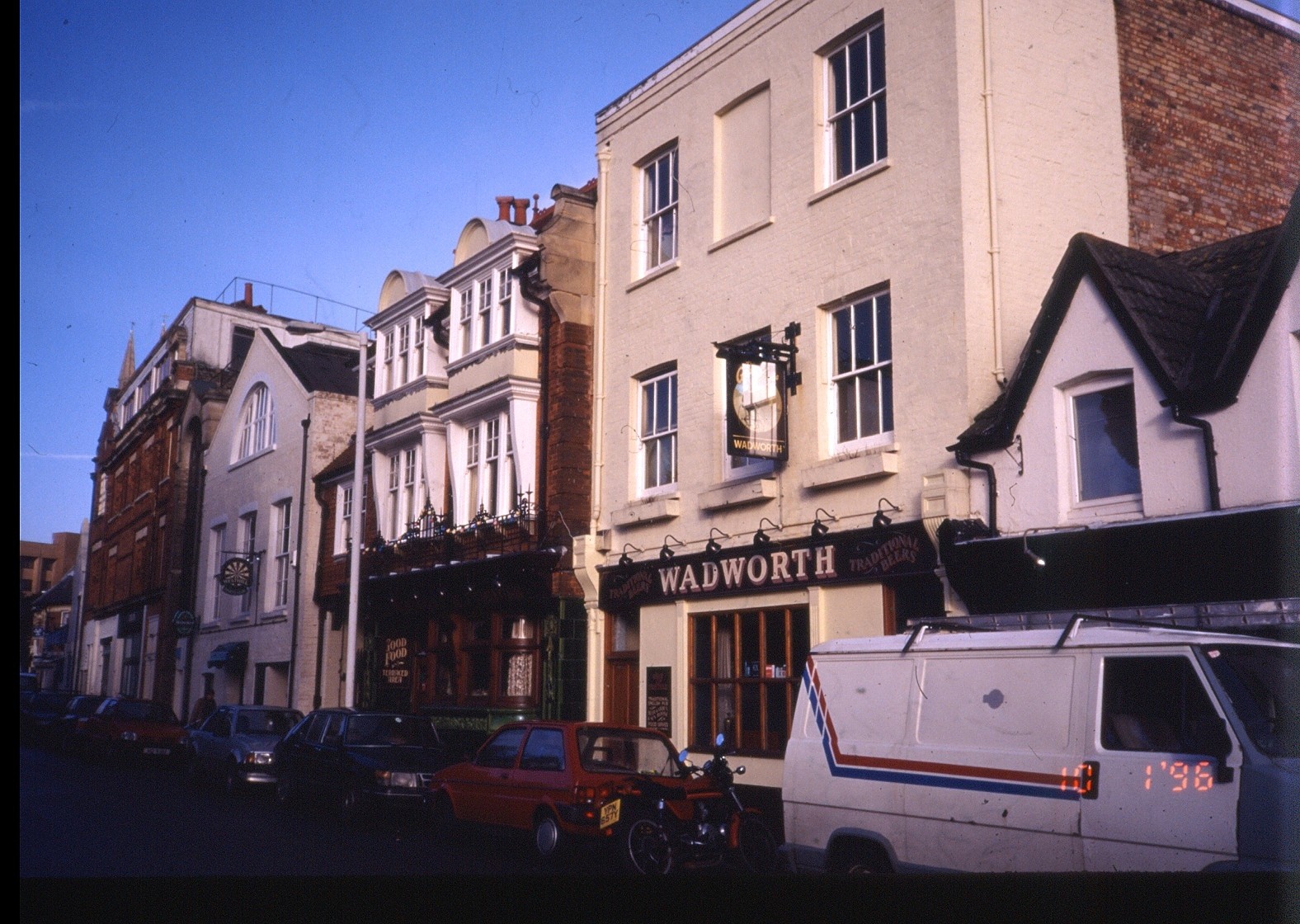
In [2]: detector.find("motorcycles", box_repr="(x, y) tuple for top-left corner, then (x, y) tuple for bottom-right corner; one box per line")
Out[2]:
(611, 730), (778, 883)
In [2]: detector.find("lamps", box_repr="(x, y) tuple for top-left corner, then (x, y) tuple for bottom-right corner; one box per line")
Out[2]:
(245, 549), (266, 562)
(706, 528), (732, 555)
(1023, 525), (1089, 566)
(812, 508), (838, 537)
(619, 543), (644, 567)
(660, 535), (687, 562)
(754, 518), (782, 545)
(873, 498), (899, 531)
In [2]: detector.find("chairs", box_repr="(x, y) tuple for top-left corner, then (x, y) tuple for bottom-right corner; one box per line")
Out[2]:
(1111, 687), (1183, 751)
(236, 715), (249, 732)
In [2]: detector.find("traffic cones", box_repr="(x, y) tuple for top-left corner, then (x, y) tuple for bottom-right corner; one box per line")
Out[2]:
(284, 317), (370, 708)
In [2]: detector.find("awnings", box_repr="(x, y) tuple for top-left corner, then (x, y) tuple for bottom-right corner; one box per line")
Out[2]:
(207, 642), (248, 667)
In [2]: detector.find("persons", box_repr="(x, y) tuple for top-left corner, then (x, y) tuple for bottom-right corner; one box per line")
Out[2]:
(190, 687), (216, 723)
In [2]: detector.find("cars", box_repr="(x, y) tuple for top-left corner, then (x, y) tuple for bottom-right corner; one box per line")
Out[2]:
(83, 693), (192, 770)
(185, 704), (314, 799)
(19, 673), (108, 754)
(271, 705), (456, 834)
(426, 719), (696, 873)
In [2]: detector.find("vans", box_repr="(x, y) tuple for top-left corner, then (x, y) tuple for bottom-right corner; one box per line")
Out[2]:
(778, 619), (1300, 923)
